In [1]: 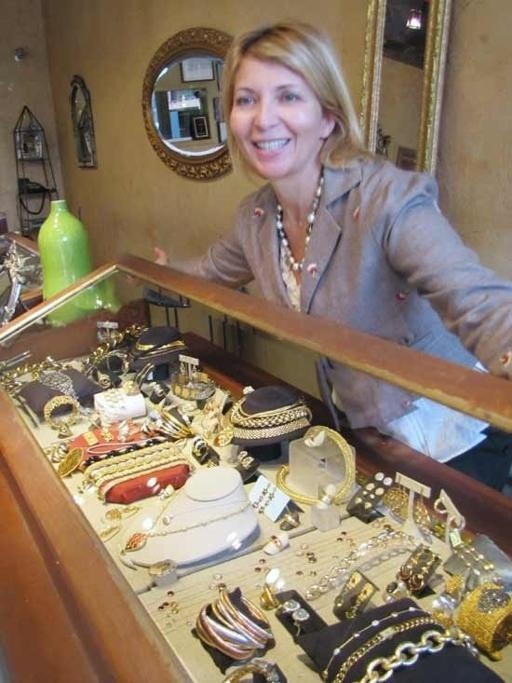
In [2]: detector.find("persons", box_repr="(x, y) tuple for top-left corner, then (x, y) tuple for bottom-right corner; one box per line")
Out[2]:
(153, 21), (512, 465)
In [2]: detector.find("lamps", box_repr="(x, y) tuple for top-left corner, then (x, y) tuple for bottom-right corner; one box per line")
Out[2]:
(406, 7), (422, 31)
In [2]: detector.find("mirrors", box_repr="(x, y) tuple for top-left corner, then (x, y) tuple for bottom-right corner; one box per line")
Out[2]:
(360, 0), (455, 177)
(142, 26), (236, 181)
(68, 75), (95, 169)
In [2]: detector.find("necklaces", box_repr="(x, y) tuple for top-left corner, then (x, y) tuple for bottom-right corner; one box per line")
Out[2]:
(1, 321), (187, 411)
(275, 172), (326, 272)
(217, 385), (315, 447)
(83, 439), (187, 491)
(310, 603), (480, 683)
(122, 481), (254, 550)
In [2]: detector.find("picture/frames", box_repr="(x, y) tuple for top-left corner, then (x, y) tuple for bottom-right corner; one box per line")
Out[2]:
(179, 55), (214, 84)
(217, 121), (226, 142)
(192, 114), (211, 142)
(216, 61), (226, 91)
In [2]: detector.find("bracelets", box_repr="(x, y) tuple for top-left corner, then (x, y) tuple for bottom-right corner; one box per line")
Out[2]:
(158, 410), (191, 439)
(191, 582), (286, 683)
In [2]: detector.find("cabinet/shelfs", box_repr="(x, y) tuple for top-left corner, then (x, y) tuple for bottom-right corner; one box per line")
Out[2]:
(14, 106), (60, 237)
(0, 254), (511, 683)
(0, 231), (46, 330)
